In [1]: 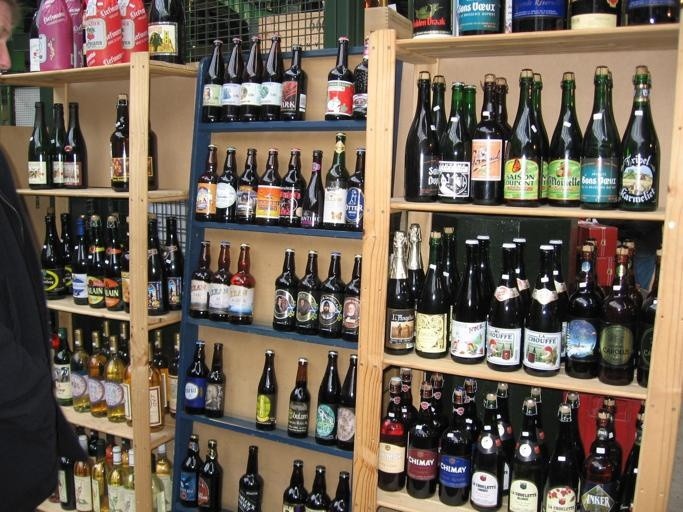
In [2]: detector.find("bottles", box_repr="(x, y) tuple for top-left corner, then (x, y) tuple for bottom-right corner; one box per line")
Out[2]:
(273, 248), (300, 332)
(207, 240), (233, 322)
(48, 103), (67, 189)
(27, 101), (53, 190)
(282, 460), (308, 512)
(522, 243), (562, 377)
(215, 146), (239, 224)
(70, 328), (90, 412)
(614, 412), (647, 512)
(336, 354), (358, 451)
(408, 223), (426, 318)
(101, 215), (122, 312)
(64, 0), (84, 68)
(51, 327), (75, 407)
(585, 237), (606, 300)
(438, 385), (475, 506)
(495, 77), (513, 153)
(322, 132), (349, 230)
(637, 248), (663, 388)
(314, 350), (341, 446)
(508, 396), (545, 512)
(470, 392), (505, 512)
(287, 357), (310, 438)
(161, 215), (184, 310)
(399, 367), (419, 476)
(59, 213), (74, 296)
(58, 454), (75, 510)
(511, 0), (568, 32)
(533, 72), (548, 139)
(103, 334), (126, 423)
(256, 349), (278, 431)
(218, 38), (244, 122)
(344, 146), (366, 231)
(167, 333), (185, 418)
(87, 331), (109, 417)
(257, 36), (285, 121)
(603, 396), (623, 480)
(625, 0), (681, 26)
(485, 242), (523, 371)
(110, 94), (131, 193)
(618, 66), (660, 212)
(325, 35), (355, 120)
(327, 471), (351, 512)
(47, 208), (63, 250)
(279, 45), (308, 120)
(101, 319), (114, 358)
(623, 239), (643, 312)
(86, 214), (105, 308)
(123, 341), (134, 426)
(406, 380), (441, 500)
(495, 382), (514, 496)
(88, 429), (100, 465)
(414, 231), (450, 359)
(567, 0), (623, 29)
(121, 438), (130, 471)
(404, 71), (439, 202)
(151, 453), (165, 512)
(463, 377), (484, 441)
(580, 65), (619, 210)
(76, 425), (89, 441)
(431, 75), (448, 142)
(456, 0), (504, 35)
(155, 443), (173, 512)
(91, 438), (112, 512)
(116, 0), (149, 63)
(565, 244), (599, 379)
(438, 81), (472, 204)
(541, 402), (577, 512)
(105, 433), (117, 471)
(194, 144), (219, 221)
(121, 216), (130, 313)
(385, 230), (415, 355)
(430, 372), (451, 484)
(352, 37), (369, 121)
(147, 342), (164, 433)
(147, 120), (159, 191)
(378, 376), (409, 492)
(548, 71), (581, 208)
(503, 69), (541, 207)
(64, 102), (88, 188)
(188, 241), (213, 319)
(441, 226), (463, 347)
(148, 0), (187, 64)
(81, 0), (127, 67)
(477, 235), (496, 315)
(123, 449), (136, 512)
(549, 239), (572, 363)
(464, 84), (477, 137)
(469, 73), (504, 206)
(576, 409), (619, 512)
(294, 250), (322, 335)
(179, 434), (204, 508)
(35, 0), (72, 72)
(608, 69), (618, 135)
(152, 328), (170, 411)
(303, 465), (331, 512)
(48, 483), (59, 503)
(204, 342), (226, 417)
(255, 148), (282, 226)
(237, 36), (263, 122)
(197, 439), (222, 512)
(565, 391), (586, 509)
(317, 252), (346, 339)
(111, 213), (124, 248)
(71, 216), (90, 305)
(234, 147), (260, 225)
(598, 245), (636, 386)
(184, 340), (210, 415)
(512, 237), (532, 321)
(530, 386), (547, 460)
(413, 0), (454, 38)
(238, 446), (264, 512)
(148, 212), (168, 316)
(40, 215), (68, 301)
(96, 439), (108, 495)
(202, 39), (225, 123)
(108, 445), (123, 512)
(118, 321), (131, 363)
(73, 434), (93, 512)
(340, 255), (362, 342)
(278, 148), (307, 227)
(450, 238), (486, 364)
(24, 8), (41, 72)
(227, 243), (256, 325)
(299, 149), (324, 228)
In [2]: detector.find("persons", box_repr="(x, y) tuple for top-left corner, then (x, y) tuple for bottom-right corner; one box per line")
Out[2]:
(0, 0), (92, 512)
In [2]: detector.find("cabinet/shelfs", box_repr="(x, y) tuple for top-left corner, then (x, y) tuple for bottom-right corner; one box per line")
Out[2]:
(0, 52), (200, 512)
(172, 46), (366, 512)
(351, 1), (683, 512)
